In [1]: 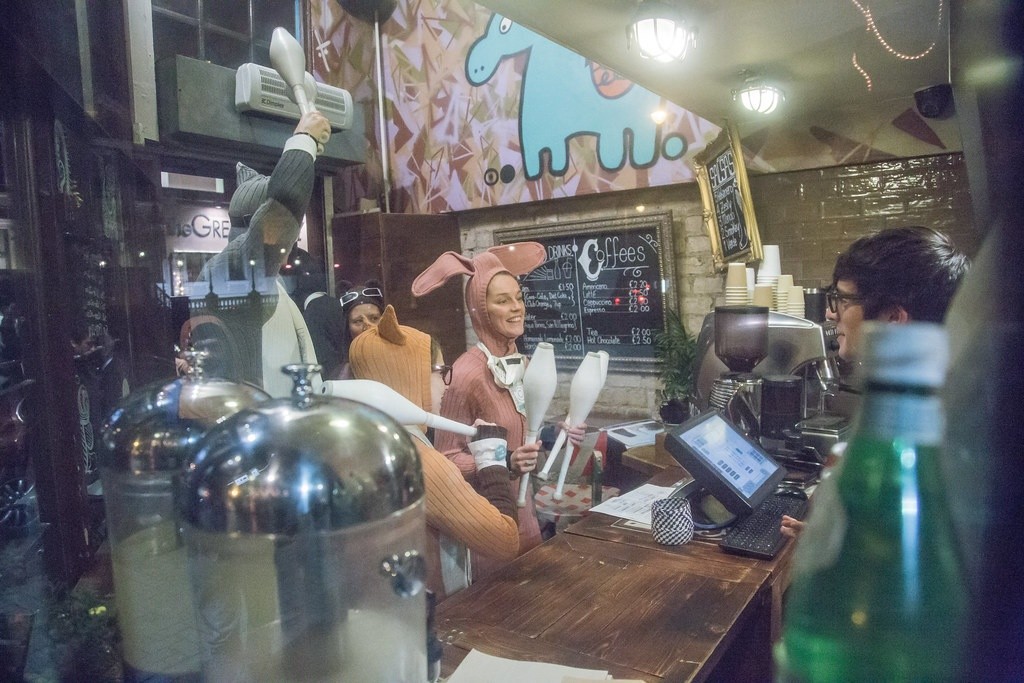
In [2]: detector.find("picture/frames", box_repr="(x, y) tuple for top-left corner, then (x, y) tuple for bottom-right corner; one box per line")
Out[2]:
(692, 123), (764, 274)
(492, 209), (680, 376)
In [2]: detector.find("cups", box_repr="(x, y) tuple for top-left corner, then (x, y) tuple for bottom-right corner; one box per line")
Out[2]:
(652, 498), (695, 546)
(723, 244), (806, 320)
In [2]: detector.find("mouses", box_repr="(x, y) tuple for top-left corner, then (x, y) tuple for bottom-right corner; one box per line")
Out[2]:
(774, 486), (807, 502)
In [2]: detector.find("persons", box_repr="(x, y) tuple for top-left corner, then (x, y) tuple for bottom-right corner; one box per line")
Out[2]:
(189, 110), (587, 683)
(779, 226), (973, 537)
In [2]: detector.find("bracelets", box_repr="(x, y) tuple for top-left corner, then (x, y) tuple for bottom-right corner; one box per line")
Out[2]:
(294, 132), (317, 145)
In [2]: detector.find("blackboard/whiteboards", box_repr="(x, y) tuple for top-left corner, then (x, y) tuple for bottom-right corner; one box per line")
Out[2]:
(693, 122), (763, 271)
(491, 207), (685, 377)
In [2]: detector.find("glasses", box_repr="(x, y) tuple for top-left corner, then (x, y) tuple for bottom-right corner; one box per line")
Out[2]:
(340, 287), (383, 311)
(826, 288), (894, 313)
(432, 366), (453, 385)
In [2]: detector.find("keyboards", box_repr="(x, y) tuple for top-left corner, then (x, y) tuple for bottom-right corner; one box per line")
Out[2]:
(718, 495), (808, 561)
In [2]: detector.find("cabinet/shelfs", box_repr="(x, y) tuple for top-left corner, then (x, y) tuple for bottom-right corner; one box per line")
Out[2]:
(0, 23), (163, 683)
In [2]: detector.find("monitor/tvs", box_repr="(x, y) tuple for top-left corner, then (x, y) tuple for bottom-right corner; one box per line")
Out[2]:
(663, 406), (788, 530)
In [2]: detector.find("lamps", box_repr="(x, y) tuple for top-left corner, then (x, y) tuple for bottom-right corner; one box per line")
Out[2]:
(731, 63), (787, 114)
(626, 0), (700, 64)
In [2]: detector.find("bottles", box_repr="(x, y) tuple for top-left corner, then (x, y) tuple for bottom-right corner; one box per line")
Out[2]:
(777, 322), (981, 683)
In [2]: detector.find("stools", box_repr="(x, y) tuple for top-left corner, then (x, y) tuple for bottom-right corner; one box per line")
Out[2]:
(534, 483), (620, 542)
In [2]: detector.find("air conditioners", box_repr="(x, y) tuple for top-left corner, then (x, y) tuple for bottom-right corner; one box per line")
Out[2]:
(234, 62), (354, 130)
